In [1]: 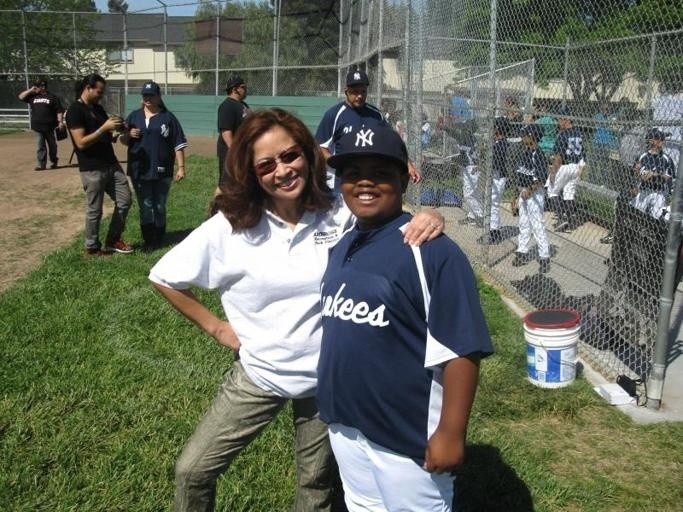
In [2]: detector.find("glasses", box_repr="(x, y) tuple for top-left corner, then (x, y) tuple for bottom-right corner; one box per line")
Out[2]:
(250, 141), (305, 176)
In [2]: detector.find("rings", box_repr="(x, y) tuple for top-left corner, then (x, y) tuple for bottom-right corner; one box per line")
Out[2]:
(431, 223), (437, 228)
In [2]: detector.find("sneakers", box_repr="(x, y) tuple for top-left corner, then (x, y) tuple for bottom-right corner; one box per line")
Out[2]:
(512, 252), (528, 265)
(600, 233), (614, 243)
(84, 248), (110, 257)
(104, 238), (133, 253)
(475, 229), (500, 245)
(554, 216), (568, 232)
(458, 216), (476, 226)
(540, 258), (550, 272)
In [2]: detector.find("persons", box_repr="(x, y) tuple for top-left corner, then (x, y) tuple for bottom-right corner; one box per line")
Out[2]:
(216, 76), (253, 200)
(380, 97), (390, 122)
(588, 103), (616, 186)
(600, 109), (642, 246)
(385, 102), (395, 126)
(394, 109), (408, 140)
(434, 117), (483, 228)
(119, 82), (188, 250)
(494, 97), (522, 142)
(317, 120), (495, 512)
(532, 100), (557, 155)
(444, 84), (476, 122)
(144, 109), (446, 510)
(652, 77), (683, 171)
(636, 128), (675, 221)
(482, 116), (508, 244)
(548, 111), (586, 231)
(65, 73), (134, 255)
(18, 75), (64, 171)
(417, 110), (432, 151)
(315, 71), (423, 186)
(511, 123), (551, 274)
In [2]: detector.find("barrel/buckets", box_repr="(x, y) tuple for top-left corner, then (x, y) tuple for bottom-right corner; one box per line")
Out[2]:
(523, 309), (582, 389)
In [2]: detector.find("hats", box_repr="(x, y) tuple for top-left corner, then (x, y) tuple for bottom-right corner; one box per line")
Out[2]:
(346, 70), (369, 85)
(645, 127), (672, 139)
(461, 119), (478, 132)
(326, 126), (408, 167)
(487, 116), (510, 132)
(223, 77), (244, 92)
(552, 110), (574, 120)
(141, 81), (160, 94)
(518, 123), (543, 136)
(33, 78), (46, 85)
